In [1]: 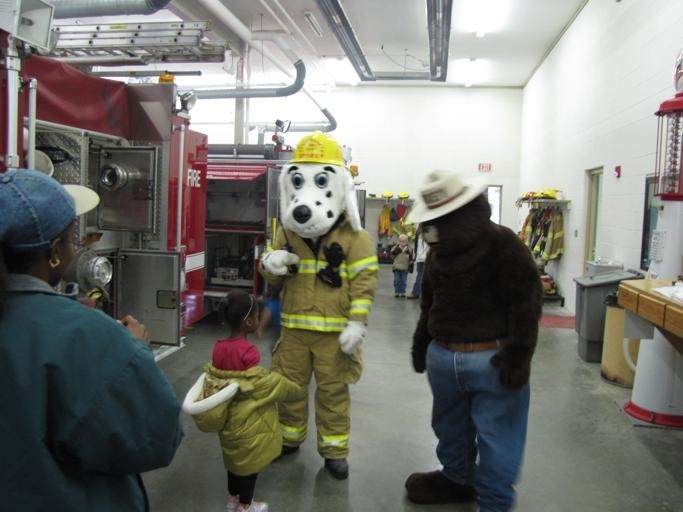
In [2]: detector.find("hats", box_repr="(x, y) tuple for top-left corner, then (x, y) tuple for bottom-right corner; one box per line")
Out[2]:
(0, 166), (101, 252)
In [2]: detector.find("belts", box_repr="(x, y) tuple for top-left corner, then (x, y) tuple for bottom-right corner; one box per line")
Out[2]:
(434, 340), (505, 351)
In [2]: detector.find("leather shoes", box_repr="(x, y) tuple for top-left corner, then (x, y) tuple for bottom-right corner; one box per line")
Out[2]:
(324, 458), (349, 479)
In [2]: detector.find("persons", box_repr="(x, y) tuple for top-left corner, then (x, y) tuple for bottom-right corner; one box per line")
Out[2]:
(252, 295), (282, 342)
(1, 168), (186, 512)
(390, 235), (414, 299)
(180, 287), (306, 511)
(405, 224), (431, 300)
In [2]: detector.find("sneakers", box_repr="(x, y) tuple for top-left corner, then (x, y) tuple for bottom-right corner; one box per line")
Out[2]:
(224, 497), (269, 512)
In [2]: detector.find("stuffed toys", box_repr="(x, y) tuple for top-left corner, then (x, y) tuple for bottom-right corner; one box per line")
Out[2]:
(402, 170), (544, 500)
(257, 131), (379, 480)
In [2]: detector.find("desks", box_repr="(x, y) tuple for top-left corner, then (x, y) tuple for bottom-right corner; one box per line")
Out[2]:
(616, 276), (683, 358)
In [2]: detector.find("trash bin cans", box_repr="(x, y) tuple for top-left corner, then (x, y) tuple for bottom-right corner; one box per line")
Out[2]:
(600, 291), (641, 388)
(572, 268), (645, 363)
